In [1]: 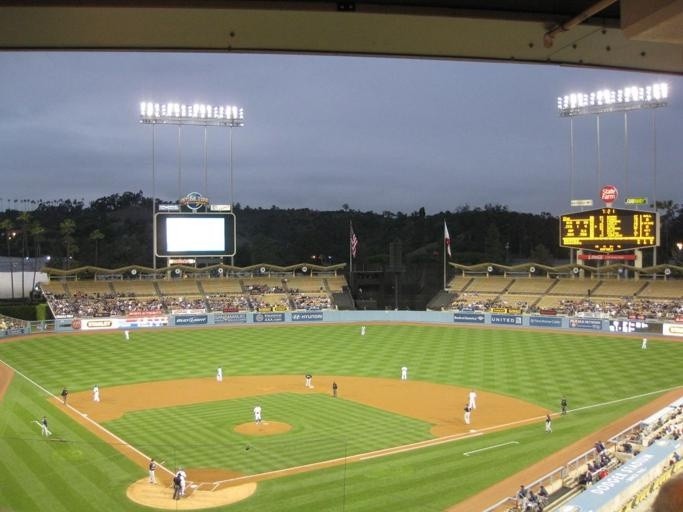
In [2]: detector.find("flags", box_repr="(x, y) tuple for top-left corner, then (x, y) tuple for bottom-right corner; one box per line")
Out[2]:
(350, 220), (358, 259)
(444, 221), (451, 257)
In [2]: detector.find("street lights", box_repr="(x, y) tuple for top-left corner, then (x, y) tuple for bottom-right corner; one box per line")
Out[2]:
(548, 78), (673, 280)
(136, 99), (246, 267)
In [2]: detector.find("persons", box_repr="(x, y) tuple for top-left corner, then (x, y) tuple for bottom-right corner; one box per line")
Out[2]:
(41, 416), (48, 436)
(172, 473), (182, 501)
(216, 364), (223, 383)
(360, 325), (365, 335)
(642, 335), (647, 349)
(448, 287), (683, 325)
(464, 403), (472, 424)
(60, 386), (69, 403)
(469, 389), (477, 408)
(401, 364), (407, 380)
(254, 403), (263, 422)
(124, 329), (130, 339)
(148, 459), (157, 484)
(175, 466), (187, 496)
(333, 380), (337, 396)
(93, 383), (100, 402)
(514, 395), (683, 512)
(45, 283), (336, 320)
(305, 370), (312, 387)
